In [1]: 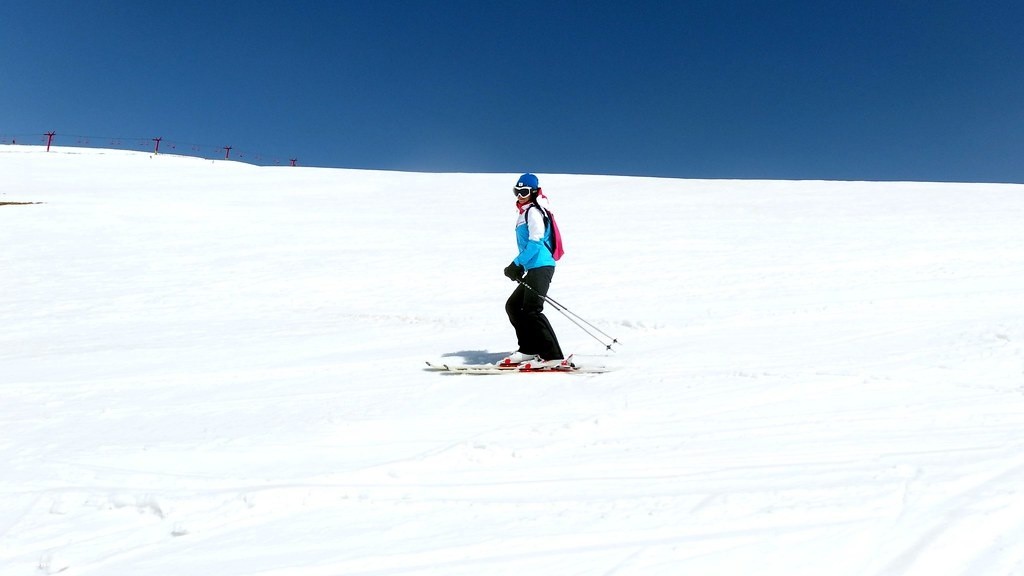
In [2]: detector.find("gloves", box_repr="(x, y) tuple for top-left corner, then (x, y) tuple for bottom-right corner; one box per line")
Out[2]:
(503, 260), (525, 283)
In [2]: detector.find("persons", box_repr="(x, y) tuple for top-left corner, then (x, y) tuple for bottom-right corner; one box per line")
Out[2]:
(495, 174), (571, 369)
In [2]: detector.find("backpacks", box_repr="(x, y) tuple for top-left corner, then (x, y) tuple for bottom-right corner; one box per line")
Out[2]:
(525, 203), (565, 261)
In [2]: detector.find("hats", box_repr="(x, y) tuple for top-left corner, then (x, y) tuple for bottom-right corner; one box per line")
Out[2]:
(517, 173), (538, 190)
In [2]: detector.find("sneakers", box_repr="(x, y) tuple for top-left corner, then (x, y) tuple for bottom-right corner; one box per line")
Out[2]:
(516, 353), (564, 370)
(495, 351), (537, 367)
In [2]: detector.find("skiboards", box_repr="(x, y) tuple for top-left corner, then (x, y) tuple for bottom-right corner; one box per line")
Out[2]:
(424, 352), (625, 376)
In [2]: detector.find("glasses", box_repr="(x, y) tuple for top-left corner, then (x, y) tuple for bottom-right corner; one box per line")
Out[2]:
(514, 186), (532, 199)
